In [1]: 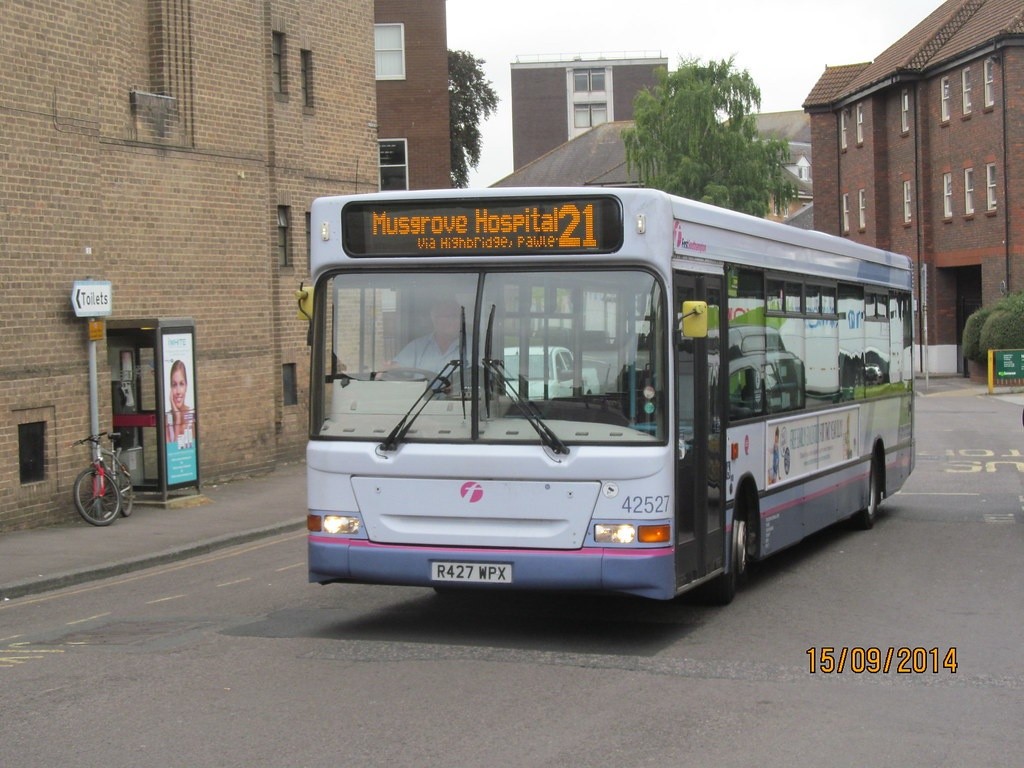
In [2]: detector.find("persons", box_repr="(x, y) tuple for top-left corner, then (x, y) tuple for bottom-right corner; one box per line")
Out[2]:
(844, 412), (854, 458)
(380, 293), (486, 397)
(163, 360), (196, 442)
(770, 425), (782, 483)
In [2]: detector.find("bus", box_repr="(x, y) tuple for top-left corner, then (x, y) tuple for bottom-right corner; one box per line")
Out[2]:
(294, 185), (916, 607)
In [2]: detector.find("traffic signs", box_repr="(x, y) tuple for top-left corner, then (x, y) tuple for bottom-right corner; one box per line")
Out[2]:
(89, 321), (104, 341)
(71, 281), (112, 318)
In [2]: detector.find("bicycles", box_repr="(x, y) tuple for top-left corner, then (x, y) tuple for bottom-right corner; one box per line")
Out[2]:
(71, 431), (134, 526)
